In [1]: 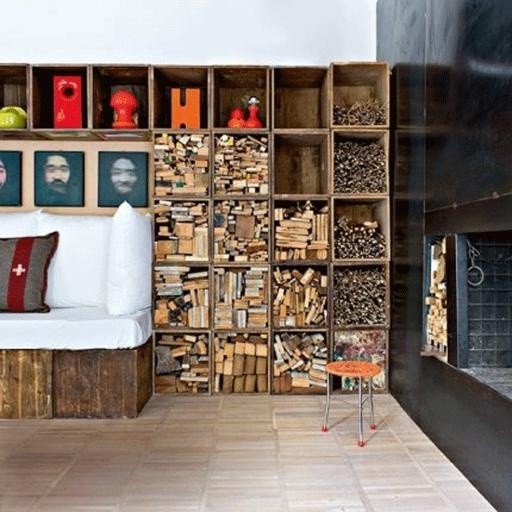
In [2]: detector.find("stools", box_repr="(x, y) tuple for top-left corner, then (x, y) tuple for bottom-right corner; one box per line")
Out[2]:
(322, 360), (382, 447)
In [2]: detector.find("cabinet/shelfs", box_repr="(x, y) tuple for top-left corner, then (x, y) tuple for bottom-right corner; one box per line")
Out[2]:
(1, 63), (152, 132)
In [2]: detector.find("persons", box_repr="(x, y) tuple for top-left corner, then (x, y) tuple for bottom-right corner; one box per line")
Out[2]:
(0, 156), (16, 204)
(39, 153), (80, 205)
(105, 156), (143, 204)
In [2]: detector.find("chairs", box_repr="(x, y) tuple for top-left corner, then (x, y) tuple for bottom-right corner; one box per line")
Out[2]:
(0, 211), (149, 419)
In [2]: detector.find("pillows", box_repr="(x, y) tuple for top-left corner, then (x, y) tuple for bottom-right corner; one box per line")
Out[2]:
(0, 230), (60, 314)
(105, 199), (151, 316)
